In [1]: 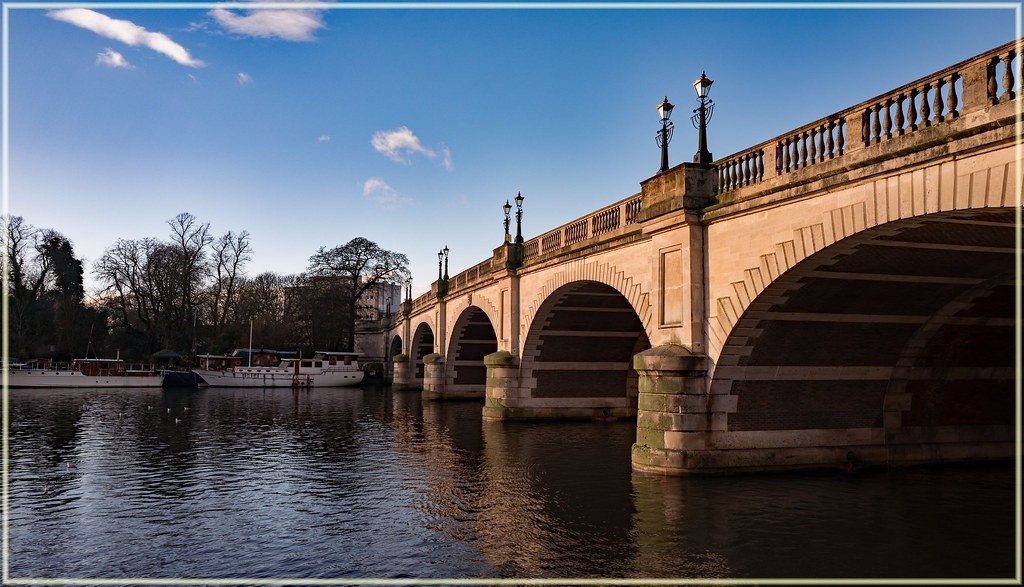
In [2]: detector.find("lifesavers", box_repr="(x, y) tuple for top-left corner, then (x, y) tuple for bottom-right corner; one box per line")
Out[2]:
(234, 359), (242, 366)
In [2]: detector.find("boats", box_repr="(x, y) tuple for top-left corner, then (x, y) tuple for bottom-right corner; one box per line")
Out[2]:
(191, 348), (367, 388)
(0, 349), (167, 387)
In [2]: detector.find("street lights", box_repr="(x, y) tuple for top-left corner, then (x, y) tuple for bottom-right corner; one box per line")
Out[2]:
(443, 245), (449, 280)
(438, 249), (443, 280)
(405, 280), (410, 302)
(408, 275), (413, 302)
(386, 298), (389, 316)
(654, 96), (676, 174)
(514, 191), (525, 244)
(248, 318), (253, 368)
(388, 296), (392, 315)
(690, 70), (715, 165)
(503, 200), (512, 244)
